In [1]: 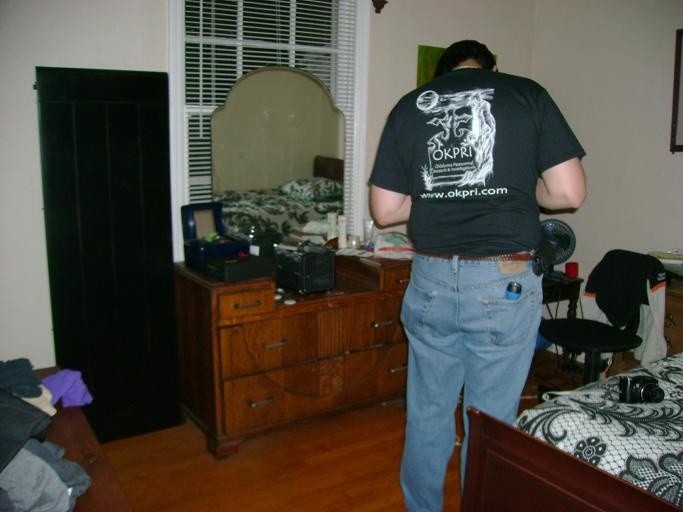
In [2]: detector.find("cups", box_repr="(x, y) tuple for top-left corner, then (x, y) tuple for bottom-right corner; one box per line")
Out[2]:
(361, 217), (374, 244)
(564, 262), (577, 279)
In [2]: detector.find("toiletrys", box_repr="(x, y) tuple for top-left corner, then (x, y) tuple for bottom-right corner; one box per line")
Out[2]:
(338, 214), (347, 248)
(363, 218), (375, 247)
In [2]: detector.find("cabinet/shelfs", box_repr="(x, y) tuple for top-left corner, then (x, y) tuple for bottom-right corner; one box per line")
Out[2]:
(171, 254), (413, 458)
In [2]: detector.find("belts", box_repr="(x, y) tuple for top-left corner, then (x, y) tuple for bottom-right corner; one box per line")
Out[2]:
(415, 250), (534, 261)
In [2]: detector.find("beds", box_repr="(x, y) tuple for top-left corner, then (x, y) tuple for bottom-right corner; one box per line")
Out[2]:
(461, 351), (682, 510)
(212, 154), (343, 246)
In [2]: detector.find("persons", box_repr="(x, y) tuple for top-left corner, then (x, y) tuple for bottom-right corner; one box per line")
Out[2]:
(366, 39), (587, 512)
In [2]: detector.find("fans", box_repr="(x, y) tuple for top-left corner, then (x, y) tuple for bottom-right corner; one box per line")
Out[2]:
(540, 220), (576, 281)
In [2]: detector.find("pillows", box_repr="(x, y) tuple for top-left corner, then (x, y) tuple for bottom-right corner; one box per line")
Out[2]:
(279, 176), (341, 202)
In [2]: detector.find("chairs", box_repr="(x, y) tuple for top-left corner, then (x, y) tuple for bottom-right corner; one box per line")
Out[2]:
(536, 248), (665, 404)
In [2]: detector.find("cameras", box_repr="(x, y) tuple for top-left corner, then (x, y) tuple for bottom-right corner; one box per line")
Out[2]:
(619, 375), (663, 403)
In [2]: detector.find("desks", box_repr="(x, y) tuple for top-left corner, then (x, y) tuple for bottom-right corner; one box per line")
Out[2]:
(539, 271), (585, 366)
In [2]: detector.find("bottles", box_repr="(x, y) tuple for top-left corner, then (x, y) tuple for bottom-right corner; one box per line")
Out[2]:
(336, 215), (347, 250)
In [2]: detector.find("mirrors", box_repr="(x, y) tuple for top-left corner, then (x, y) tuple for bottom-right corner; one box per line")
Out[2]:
(207, 64), (346, 248)
